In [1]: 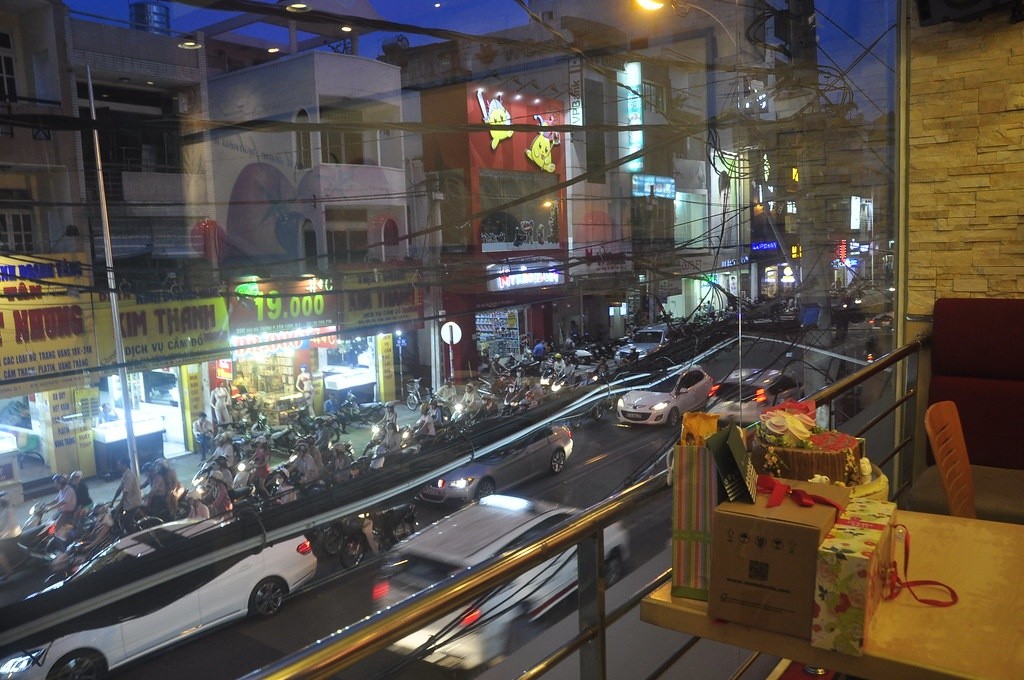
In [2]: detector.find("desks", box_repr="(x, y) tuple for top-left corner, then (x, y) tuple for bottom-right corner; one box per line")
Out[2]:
(360, 402), (384, 423)
(640, 510), (1024, 680)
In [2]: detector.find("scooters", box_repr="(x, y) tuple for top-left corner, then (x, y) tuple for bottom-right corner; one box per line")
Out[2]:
(15, 347), (610, 590)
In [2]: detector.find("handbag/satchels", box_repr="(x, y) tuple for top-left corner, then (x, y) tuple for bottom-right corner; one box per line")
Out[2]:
(666, 438), (718, 600)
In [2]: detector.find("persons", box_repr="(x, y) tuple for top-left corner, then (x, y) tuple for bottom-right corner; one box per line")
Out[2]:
(99, 404), (117, 423)
(296, 364), (315, 416)
(0, 457), (142, 582)
(694, 301), (714, 328)
(489, 320), (638, 412)
(780, 297), (794, 308)
(270, 391), (378, 554)
(374, 378), (497, 453)
(731, 277), (736, 294)
(211, 379), (233, 430)
(140, 412), (271, 521)
(836, 278), (841, 289)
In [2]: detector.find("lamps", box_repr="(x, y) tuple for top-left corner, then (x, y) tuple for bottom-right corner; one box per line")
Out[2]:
(286, 4), (311, 13)
(341, 23), (352, 32)
(177, 41), (202, 50)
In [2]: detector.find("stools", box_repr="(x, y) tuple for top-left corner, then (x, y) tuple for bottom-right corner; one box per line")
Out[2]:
(194, 442), (211, 455)
(357, 413), (373, 429)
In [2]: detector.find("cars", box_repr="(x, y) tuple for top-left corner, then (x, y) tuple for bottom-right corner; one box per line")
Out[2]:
(871, 314), (894, 331)
(772, 290), (850, 328)
(413, 417), (574, 505)
(706, 368), (804, 427)
(615, 364), (713, 427)
(614, 322), (686, 366)
(0, 515), (318, 680)
(374, 495), (629, 669)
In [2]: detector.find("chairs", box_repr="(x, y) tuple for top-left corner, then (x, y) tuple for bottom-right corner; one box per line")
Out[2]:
(924, 401), (975, 518)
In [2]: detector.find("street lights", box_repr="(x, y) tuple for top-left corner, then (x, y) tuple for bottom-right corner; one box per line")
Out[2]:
(640, 1), (840, 430)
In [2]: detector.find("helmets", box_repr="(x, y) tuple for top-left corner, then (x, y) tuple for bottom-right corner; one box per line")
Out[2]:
(53, 473), (68, 484)
(155, 457), (168, 469)
(208, 354), (606, 482)
(187, 491), (202, 501)
(141, 462), (155, 472)
(70, 471), (83, 479)
(91, 505), (108, 516)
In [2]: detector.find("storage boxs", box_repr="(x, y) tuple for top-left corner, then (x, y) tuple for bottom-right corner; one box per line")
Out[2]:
(812, 497), (897, 656)
(708, 478), (850, 639)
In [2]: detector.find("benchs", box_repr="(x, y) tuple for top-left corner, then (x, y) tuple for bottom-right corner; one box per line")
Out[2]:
(8, 431), (45, 469)
(905, 298), (1024, 524)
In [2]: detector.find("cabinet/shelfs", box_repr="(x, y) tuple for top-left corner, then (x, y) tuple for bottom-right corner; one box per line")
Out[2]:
(261, 390), (310, 425)
